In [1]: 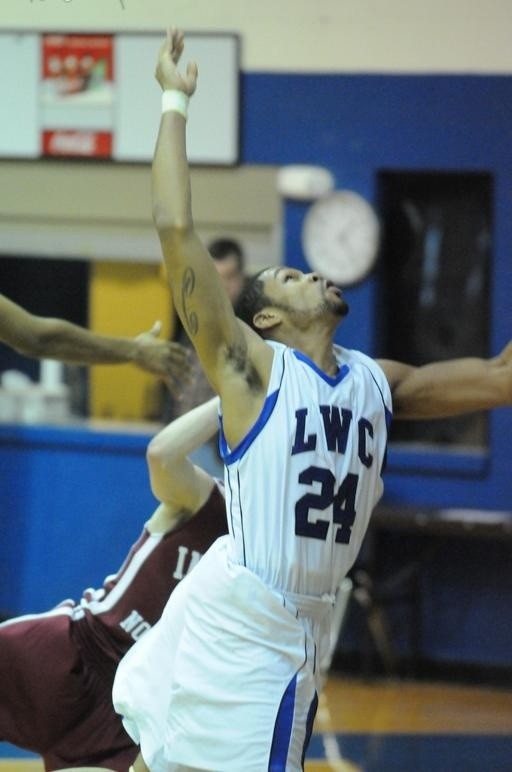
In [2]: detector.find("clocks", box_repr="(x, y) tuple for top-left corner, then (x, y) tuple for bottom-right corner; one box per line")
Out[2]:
(299, 186), (382, 287)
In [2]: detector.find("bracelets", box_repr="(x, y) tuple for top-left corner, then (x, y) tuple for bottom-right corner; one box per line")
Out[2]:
(161, 89), (190, 120)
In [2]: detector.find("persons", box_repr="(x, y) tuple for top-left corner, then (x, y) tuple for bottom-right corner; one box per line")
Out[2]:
(109, 25), (512, 772)
(1, 395), (237, 772)
(0, 291), (201, 397)
(169, 236), (255, 419)
